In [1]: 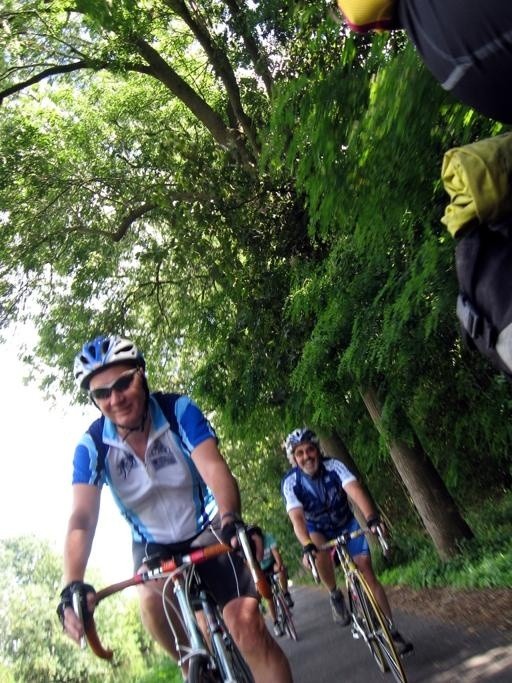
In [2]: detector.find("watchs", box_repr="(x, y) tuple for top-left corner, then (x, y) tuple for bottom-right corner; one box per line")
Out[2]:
(219, 510), (241, 521)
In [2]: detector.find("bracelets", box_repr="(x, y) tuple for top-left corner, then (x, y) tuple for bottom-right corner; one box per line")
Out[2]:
(365, 511), (378, 521)
(303, 539), (312, 545)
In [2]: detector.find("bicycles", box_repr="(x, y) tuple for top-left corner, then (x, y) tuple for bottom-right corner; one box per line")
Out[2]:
(259, 565), (301, 643)
(304, 522), (411, 682)
(70, 518), (275, 682)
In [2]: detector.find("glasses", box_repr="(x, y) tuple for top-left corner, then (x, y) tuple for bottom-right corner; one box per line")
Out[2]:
(89, 367), (138, 399)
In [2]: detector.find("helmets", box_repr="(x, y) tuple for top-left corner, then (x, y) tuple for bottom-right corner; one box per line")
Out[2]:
(74, 336), (145, 389)
(285, 428), (321, 468)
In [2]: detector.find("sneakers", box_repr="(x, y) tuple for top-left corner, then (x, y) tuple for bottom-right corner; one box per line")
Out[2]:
(329, 589), (350, 627)
(385, 629), (418, 656)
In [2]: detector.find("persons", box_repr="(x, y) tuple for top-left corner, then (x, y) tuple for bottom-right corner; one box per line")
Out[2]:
(55, 335), (294, 683)
(240, 524), (293, 637)
(280, 428), (416, 660)
